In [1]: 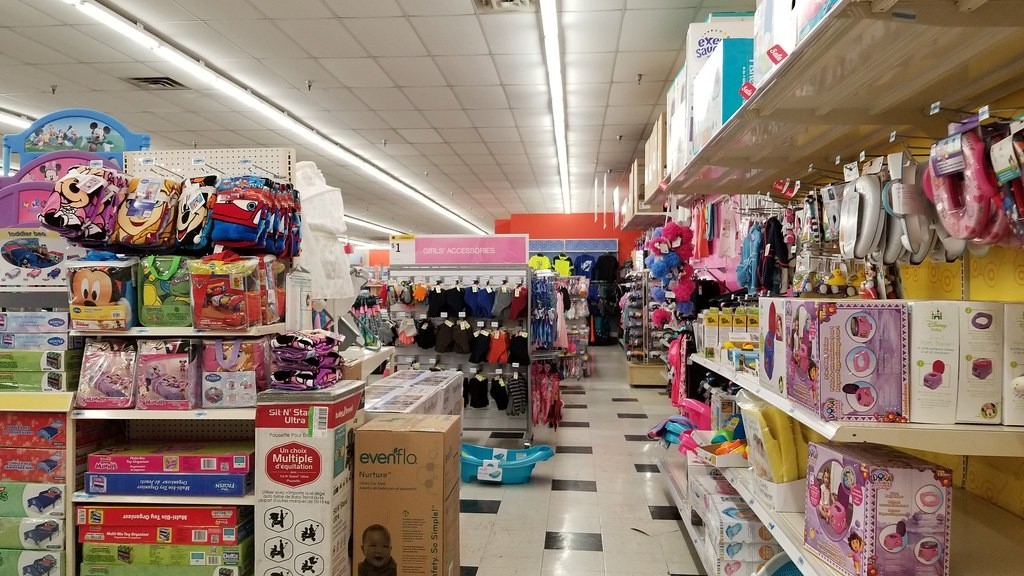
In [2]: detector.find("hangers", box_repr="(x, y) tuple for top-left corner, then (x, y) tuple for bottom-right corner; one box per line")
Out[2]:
(536, 249), (619, 306)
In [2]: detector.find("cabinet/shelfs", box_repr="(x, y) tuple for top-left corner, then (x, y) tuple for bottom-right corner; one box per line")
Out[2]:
(661, 0), (1024, 576)
(0, 271), (395, 576)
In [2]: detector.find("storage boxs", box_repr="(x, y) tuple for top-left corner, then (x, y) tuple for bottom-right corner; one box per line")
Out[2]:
(0, 307), (465, 576)
(0, 228), (67, 286)
(620, 1), (1024, 576)
